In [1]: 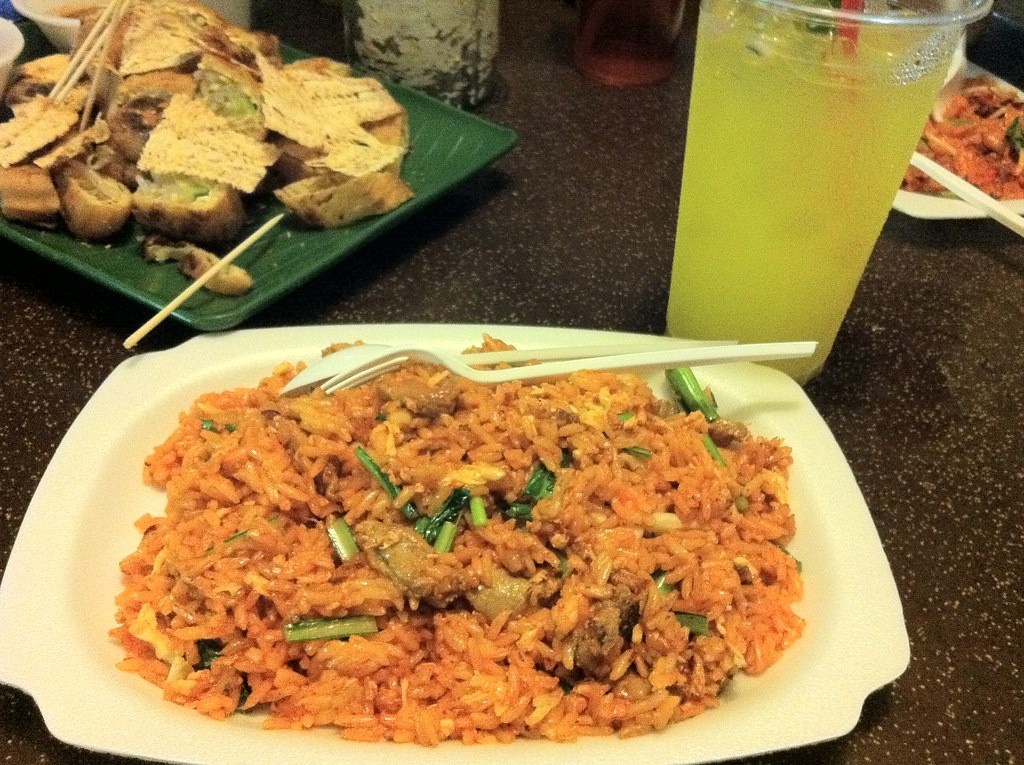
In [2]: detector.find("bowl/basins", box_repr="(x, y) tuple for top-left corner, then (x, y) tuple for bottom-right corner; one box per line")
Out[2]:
(0, 13), (25, 97)
(9, 0), (252, 53)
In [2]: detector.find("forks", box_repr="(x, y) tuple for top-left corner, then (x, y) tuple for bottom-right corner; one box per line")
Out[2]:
(320, 341), (818, 397)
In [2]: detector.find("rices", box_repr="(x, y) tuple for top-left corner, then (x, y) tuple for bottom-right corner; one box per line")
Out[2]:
(112, 333), (805, 746)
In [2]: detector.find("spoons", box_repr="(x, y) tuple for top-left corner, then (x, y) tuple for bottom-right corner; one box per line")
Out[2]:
(277, 342), (740, 397)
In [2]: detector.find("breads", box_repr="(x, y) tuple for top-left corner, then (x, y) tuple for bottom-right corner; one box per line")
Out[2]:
(0, 1), (409, 297)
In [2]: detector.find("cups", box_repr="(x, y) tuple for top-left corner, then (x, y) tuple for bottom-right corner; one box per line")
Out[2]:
(356, 0), (500, 113)
(572, 0), (687, 90)
(665, 0), (992, 387)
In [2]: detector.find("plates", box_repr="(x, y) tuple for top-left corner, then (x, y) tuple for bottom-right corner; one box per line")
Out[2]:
(0, 19), (521, 331)
(894, 59), (1024, 219)
(0, 323), (910, 765)
